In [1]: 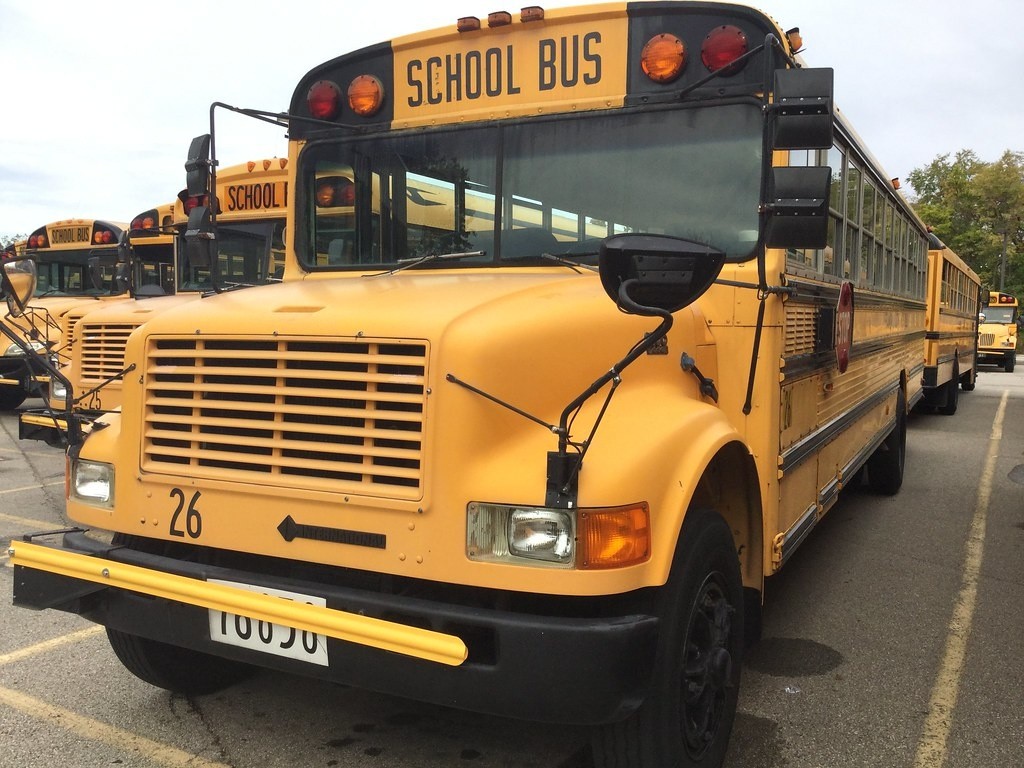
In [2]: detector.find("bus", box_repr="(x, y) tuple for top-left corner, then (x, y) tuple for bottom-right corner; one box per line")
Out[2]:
(925, 235), (991, 415)
(1, 154), (640, 454)
(974, 291), (1023, 372)
(6, 0), (930, 766)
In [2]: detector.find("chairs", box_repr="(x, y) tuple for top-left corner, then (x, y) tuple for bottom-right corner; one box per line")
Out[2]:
(270, 268), (283, 283)
(199, 275), (246, 290)
(435, 228), (557, 266)
(664, 216), (738, 259)
